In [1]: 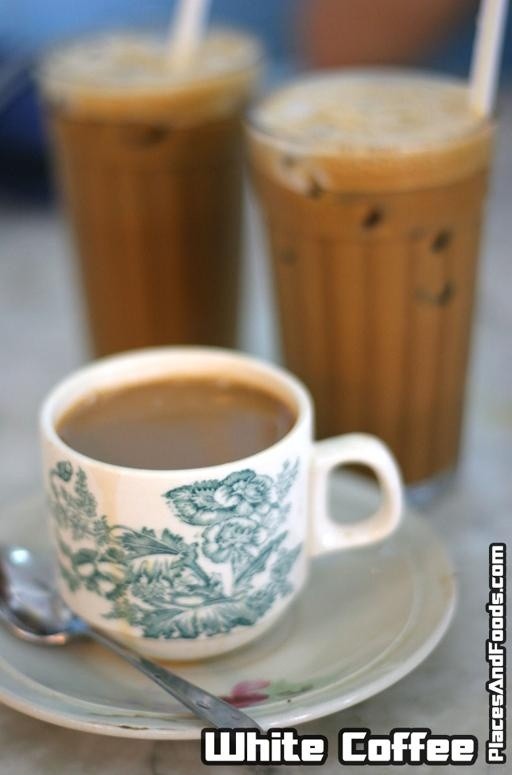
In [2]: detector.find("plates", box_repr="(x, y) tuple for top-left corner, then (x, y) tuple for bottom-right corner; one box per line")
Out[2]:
(1, 469), (456, 742)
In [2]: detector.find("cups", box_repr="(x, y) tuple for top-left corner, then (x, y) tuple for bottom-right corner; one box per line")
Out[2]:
(240, 68), (496, 481)
(37, 29), (251, 362)
(40, 345), (404, 666)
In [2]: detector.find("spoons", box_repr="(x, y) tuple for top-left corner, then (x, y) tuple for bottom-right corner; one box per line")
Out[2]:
(1, 544), (273, 737)
